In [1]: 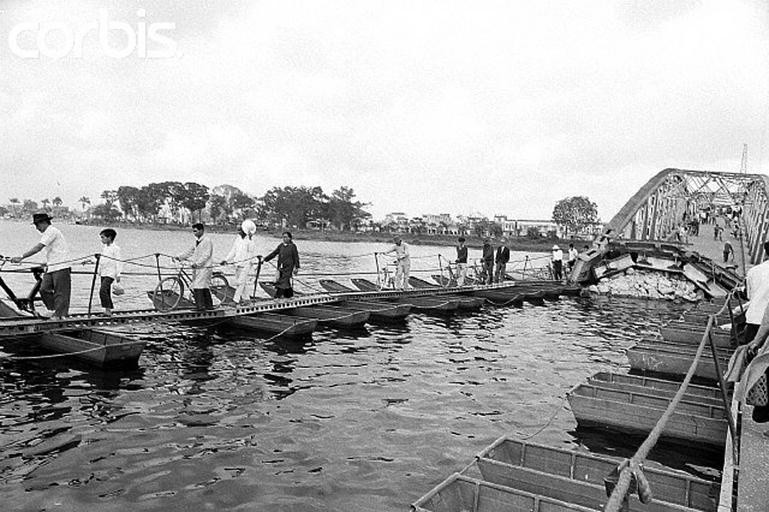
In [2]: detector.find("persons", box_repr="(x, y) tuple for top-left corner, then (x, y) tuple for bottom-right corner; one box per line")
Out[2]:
(567, 244), (577, 270)
(219, 223), (256, 308)
(492, 238), (510, 286)
(261, 232), (300, 299)
(479, 237), (494, 287)
(81, 228), (122, 316)
(172, 222), (214, 311)
(9, 212), (73, 321)
(375, 236), (411, 290)
(734, 240), (769, 442)
(455, 237), (468, 288)
(551, 244), (564, 281)
(577, 245), (589, 261)
(672, 201), (743, 261)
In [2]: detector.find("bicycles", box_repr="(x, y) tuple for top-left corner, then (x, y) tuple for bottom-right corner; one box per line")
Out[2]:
(440, 257), (491, 288)
(153, 256), (229, 313)
(0, 254), (57, 320)
(375, 252), (405, 291)
(729, 250), (734, 262)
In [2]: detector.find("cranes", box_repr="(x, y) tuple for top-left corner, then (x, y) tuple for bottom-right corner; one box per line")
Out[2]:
(717, 229), (724, 241)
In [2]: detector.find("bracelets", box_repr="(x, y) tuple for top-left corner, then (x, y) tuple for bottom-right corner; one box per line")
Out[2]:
(20, 255), (23, 259)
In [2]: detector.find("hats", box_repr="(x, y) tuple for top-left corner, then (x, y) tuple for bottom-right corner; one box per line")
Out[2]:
(241, 218), (257, 235)
(30, 212), (54, 225)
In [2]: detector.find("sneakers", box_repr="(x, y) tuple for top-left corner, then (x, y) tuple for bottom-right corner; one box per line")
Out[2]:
(189, 305), (215, 311)
(228, 296), (296, 308)
